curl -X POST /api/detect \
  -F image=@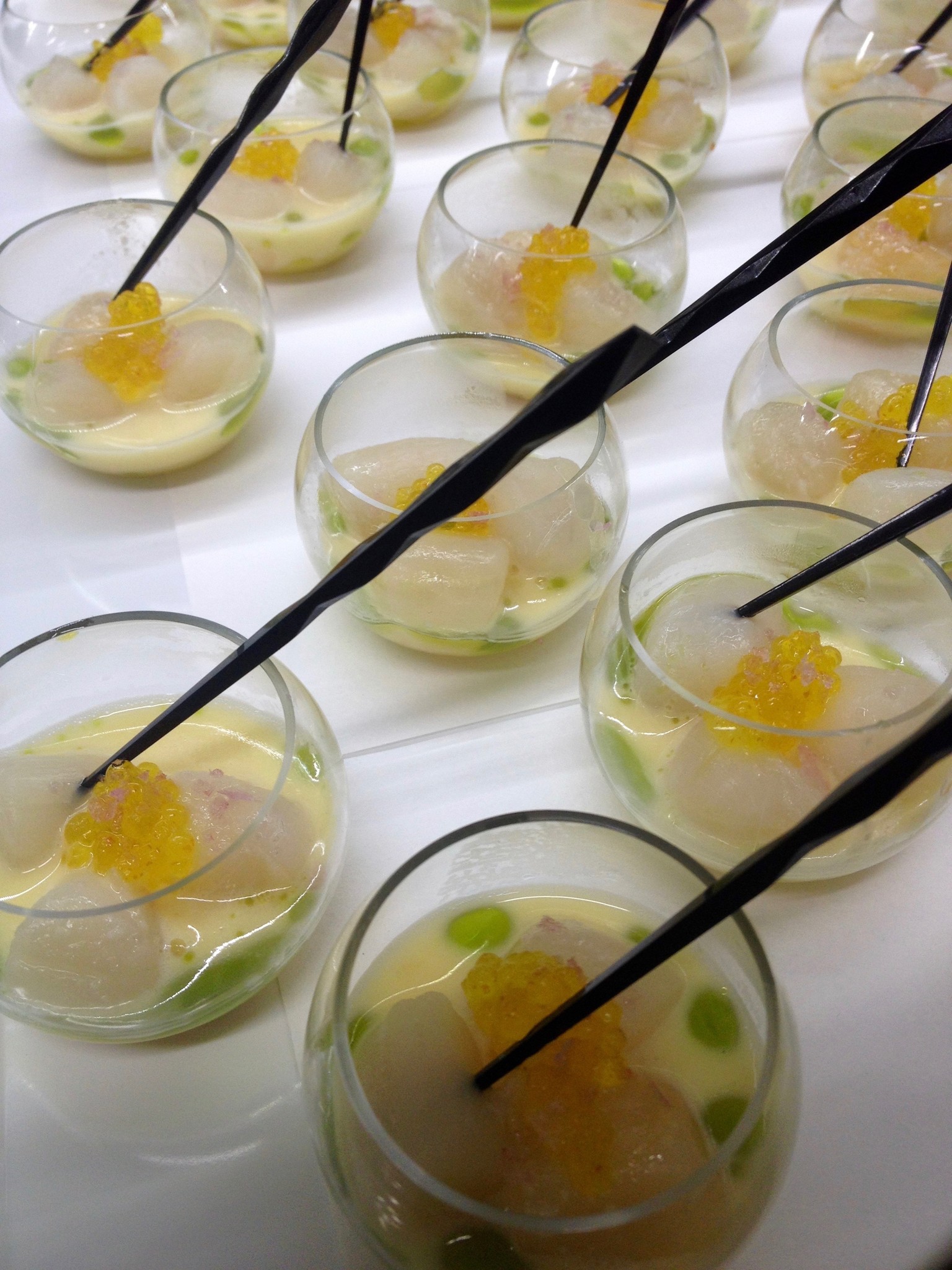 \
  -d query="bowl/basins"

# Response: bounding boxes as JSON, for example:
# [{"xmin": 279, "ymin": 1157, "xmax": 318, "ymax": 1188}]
[
  {"xmin": 417, "ymin": 139, "xmax": 688, "ymax": 400},
  {"xmin": 800, "ymin": 0, "xmax": 952, "ymax": 130},
  {"xmin": 781, "ymin": 96, "xmax": 952, "ymax": 344},
  {"xmin": 581, "ymin": 499, "xmax": 952, "ymax": 882},
  {"xmin": 0, "ymin": 0, "xmax": 209, "ymax": 165},
  {"xmin": 721, "ymin": 278, "xmax": 952, "ymax": 590},
  {"xmin": 303, "ymin": 809, "xmax": 802, "ymax": 1269},
  {"xmin": 285, "ymin": 0, "xmax": 490, "ymax": 132},
  {"xmin": 0, "ymin": 611, "xmax": 348, "ymax": 1043},
  {"xmin": 490, "ymin": 0, "xmax": 781, "ymax": 207},
  {"xmin": 0, "ymin": 199, "xmax": 276, "ymax": 479},
  {"xmin": 151, "ymin": 43, "xmax": 395, "ymax": 274},
  {"xmin": 293, "ymin": 332, "xmax": 629, "ymax": 657},
  {"xmin": 179, "ymin": 0, "xmax": 290, "ymax": 48}
]
